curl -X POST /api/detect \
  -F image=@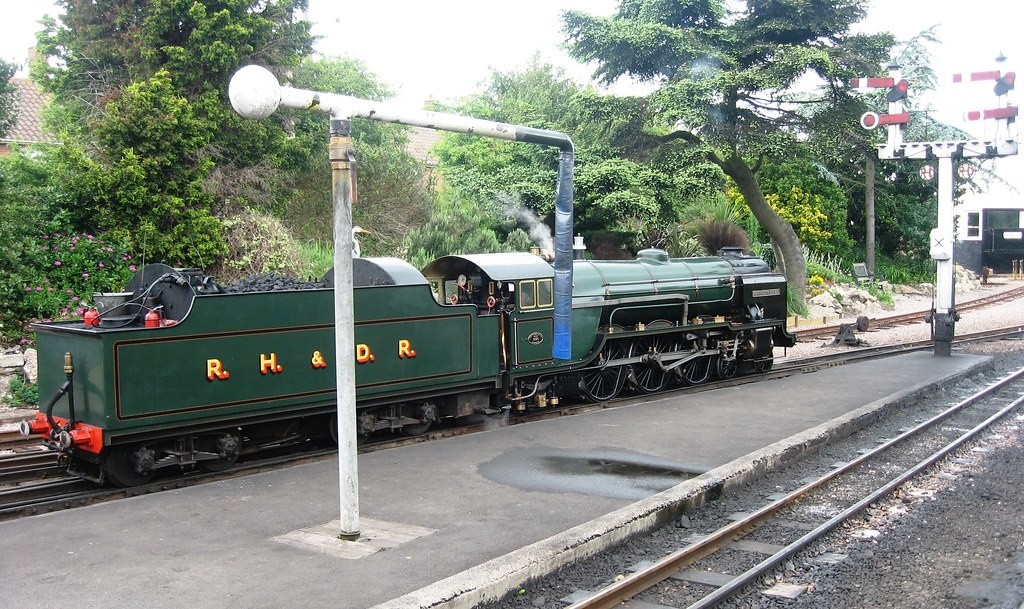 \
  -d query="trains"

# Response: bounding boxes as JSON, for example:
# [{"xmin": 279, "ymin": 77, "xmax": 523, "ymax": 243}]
[{"xmin": 20, "ymin": 224, "xmax": 798, "ymax": 492}]
